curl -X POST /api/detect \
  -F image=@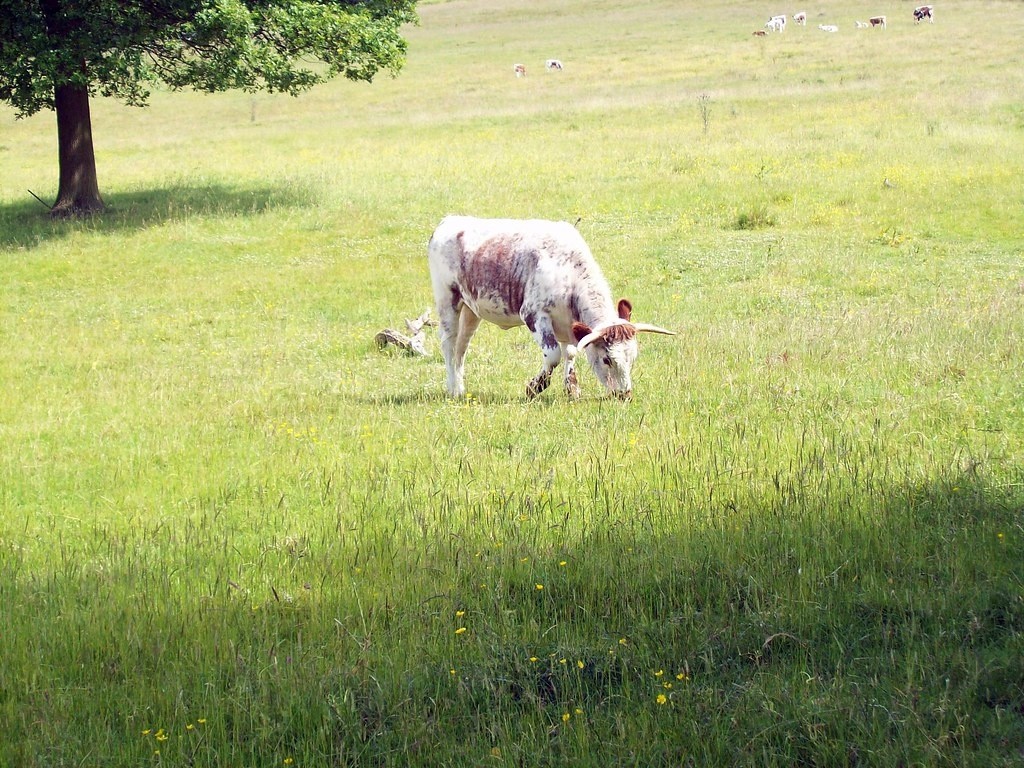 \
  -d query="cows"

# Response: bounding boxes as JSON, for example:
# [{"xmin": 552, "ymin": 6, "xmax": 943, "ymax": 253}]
[
  {"xmin": 792, "ymin": 12, "xmax": 806, "ymax": 26},
  {"xmin": 752, "ymin": 30, "xmax": 767, "ymax": 37},
  {"xmin": 912, "ymin": 5, "xmax": 934, "ymax": 26},
  {"xmin": 869, "ymin": 16, "xmax": 887, "ymax": 31},
  {"xmin": 854, "ymin": 21, "xmax": 869, "ymax": 29},
  {"xmin": 764, "ymin": 15, "xmax": 786, "ymax": 33},
  {"xmin": 816, "ymin": 23, "xmax": 839, "ymax": 32},
  {"xmin": 514, "ymin": 63, "xmax": 528, "ymax": 79},
  {"xmin": 428, "ymin": 214, "xmax": 676, "ymax": 406},
  {"xmin": 546, "ymin": 60, "xmax": 563, "ymax": 72}
]
